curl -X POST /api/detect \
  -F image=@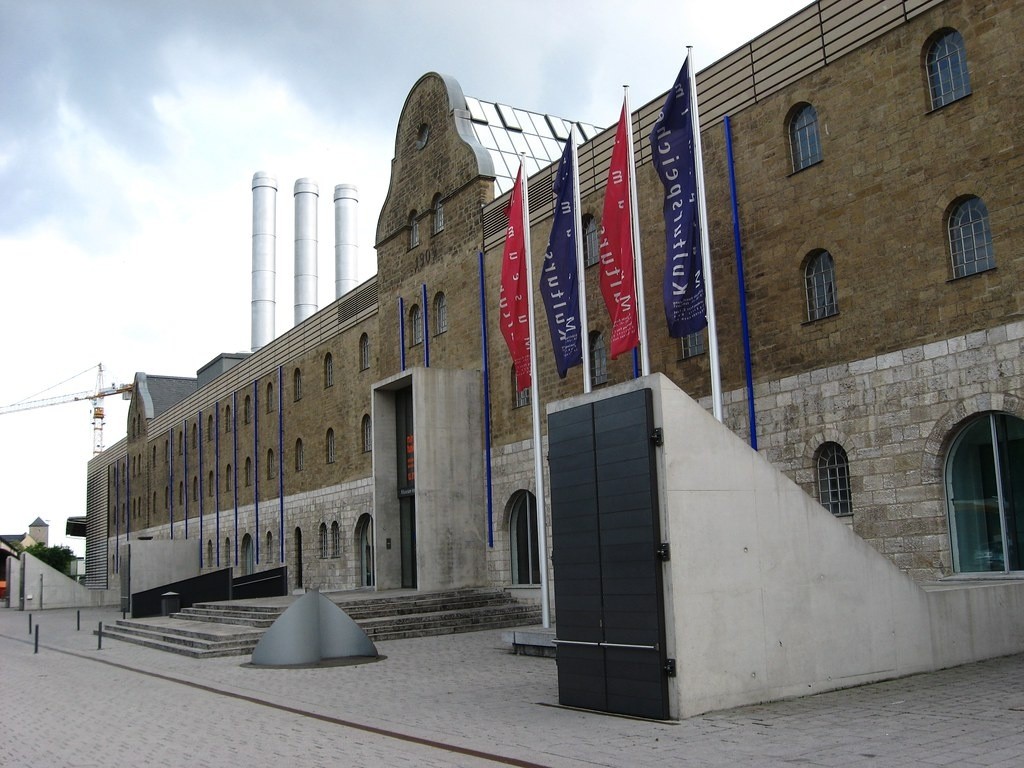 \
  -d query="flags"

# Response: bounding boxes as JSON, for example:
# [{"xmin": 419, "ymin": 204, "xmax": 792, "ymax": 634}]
[
  {"xmin": 648, "ymin": 55, "xmax": 713, "ymax": 333},
  {"xmin": 598, "ymin": 95, "xmax": 646, "ymax": 361},
  {"xmin": 498, "ymin": 154, "xmax": 536, "ymax": 393},
  {"xmin": 539, "ymin": 123, "xmax": 584, "ymax": 380}
]
